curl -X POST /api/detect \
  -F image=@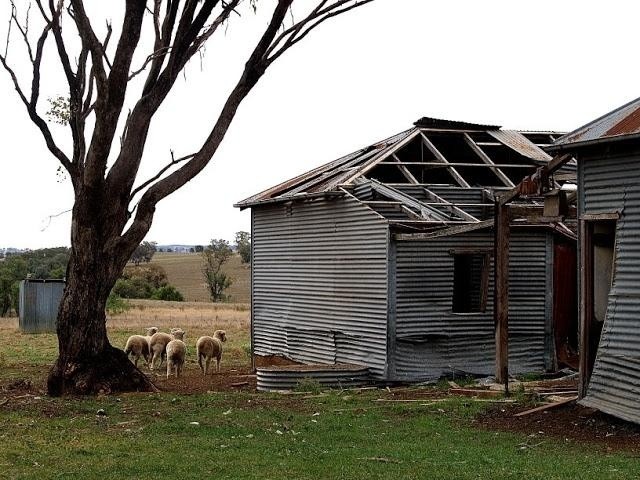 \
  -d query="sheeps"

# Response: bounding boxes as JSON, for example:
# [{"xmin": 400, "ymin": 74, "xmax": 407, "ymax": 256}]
[
  {"xmin": 165, "ymin": 330, "xmax": 187, "ymax": 380},
  {"xmin": 147, "ymin": 328, "xmax": 183, "ymax": 370},
  {"xmin": 124, "ymin": 326, "xmax": 159, "ymax": 368},
  {"xmin": 196, "ymin": 330, "xmax": 228, "ymax": 376}
]
